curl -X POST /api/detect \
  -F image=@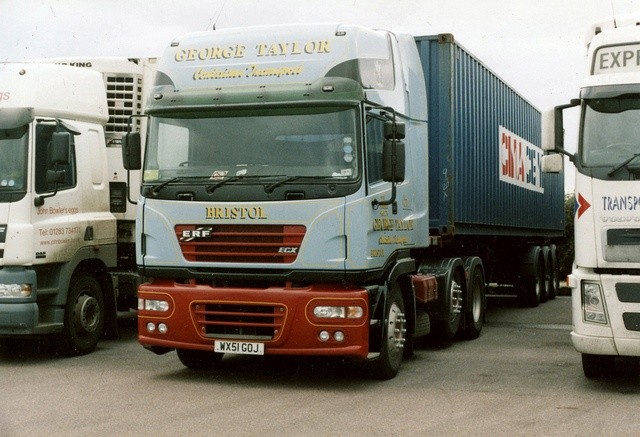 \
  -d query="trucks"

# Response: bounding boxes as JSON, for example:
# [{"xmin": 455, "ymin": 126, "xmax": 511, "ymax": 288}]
[
  {"xmin": 1, "ymin": 56, "xmax": 146, "ymax": 357},
  {"xmin": 121, "ymin": 22, "xmax": 565, "ymax": 381},
  {"xmin": 542, "ymin": 26, "xmax": 640, "ymax": 384}
]
